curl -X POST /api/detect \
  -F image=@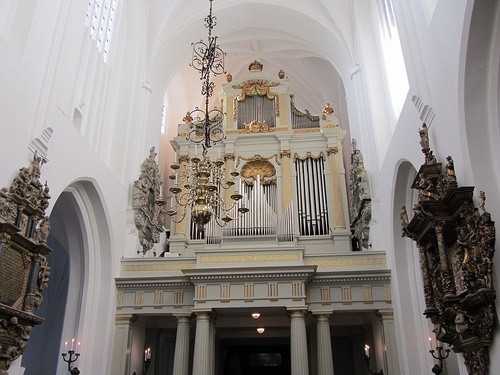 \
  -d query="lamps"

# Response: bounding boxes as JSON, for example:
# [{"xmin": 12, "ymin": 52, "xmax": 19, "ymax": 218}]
[
  {"xmin": 252, "ymin": 312, "xmax": 261, "ymax": 320},
  {"xmin": 364, "ymin": 343, "xmax": 383, "ymax": 375},
  {"xmin": 427, "ymin": 330, "xmax": 452, "ymax": 375},
  {"xmin": 157, "ymin": 0, "xmax": 250, "ymax": 229},
  {"xmin": 256, "ymin": 327, "xmax": 265, "ymax": 335},
  {"xmin": 61, "ymin": 337, "xmax": 81, "ymax": 375},
  {"xmin": 132, "ymin": 347, "xmax": 153, "ymax": 375}
]
